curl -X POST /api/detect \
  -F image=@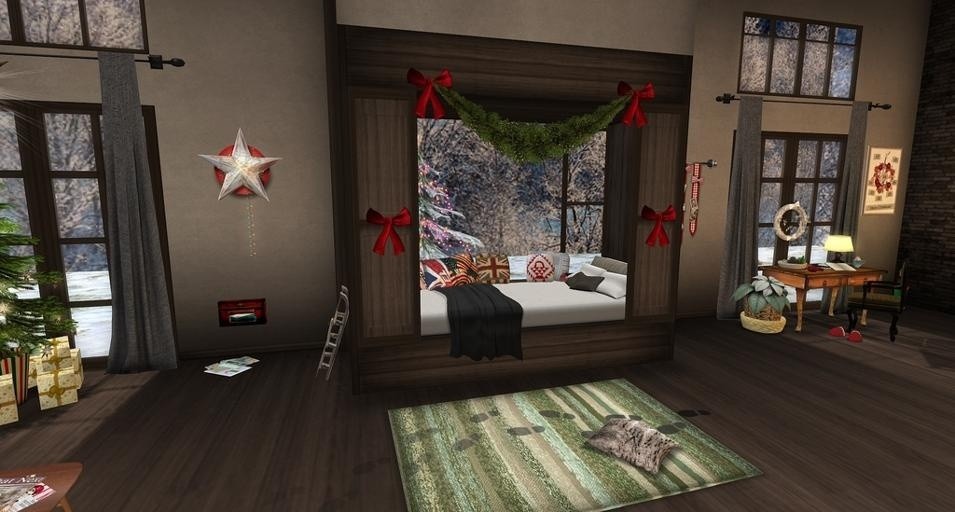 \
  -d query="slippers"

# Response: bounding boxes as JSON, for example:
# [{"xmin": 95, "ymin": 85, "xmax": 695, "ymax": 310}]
[
  {"xmin": 829, "ymin": 326, "xmax": 846, "ymax": 337},
  {"xmin": 848, "ymin": 330, "xmax": 863, "ymax": 343}
]
